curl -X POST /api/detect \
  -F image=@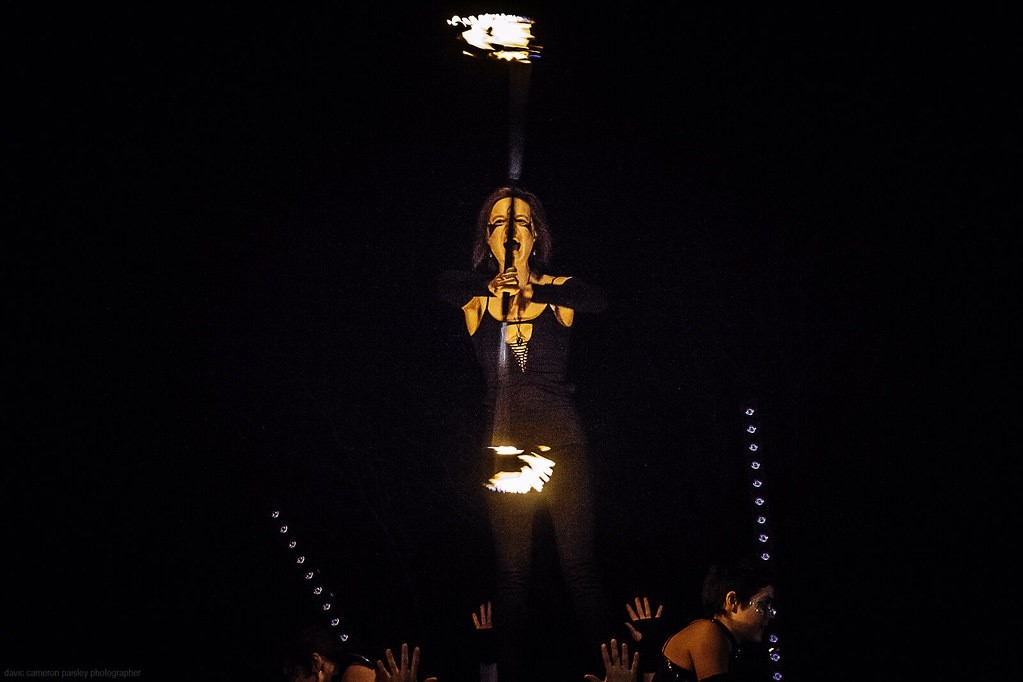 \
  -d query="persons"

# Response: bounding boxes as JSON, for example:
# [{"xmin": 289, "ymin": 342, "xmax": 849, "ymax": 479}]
[
  {"xmin": 282, "ymin": 626, "xmax": 383, "ymax": 682},
  {"xmin": 584, "ymin": 638, "xmax": 640, "ymax": 682},
  {"xmin": 651, "ymin": 556, "xmax": 785, "ymax": 682},
  {"xmin": 470, "ymin": 600, "xmax": 501, "ymax": 682},
  {"xmin": 376, "ymin": 643, "xmax": 438, "ymax": 682},
  {"xmin": 440, "ymin": 185, "xmax": 608, "ymax": 682},
  {"xmin": 624, "ymin": 593, "xmax": 665, "ymax": 682}
]
